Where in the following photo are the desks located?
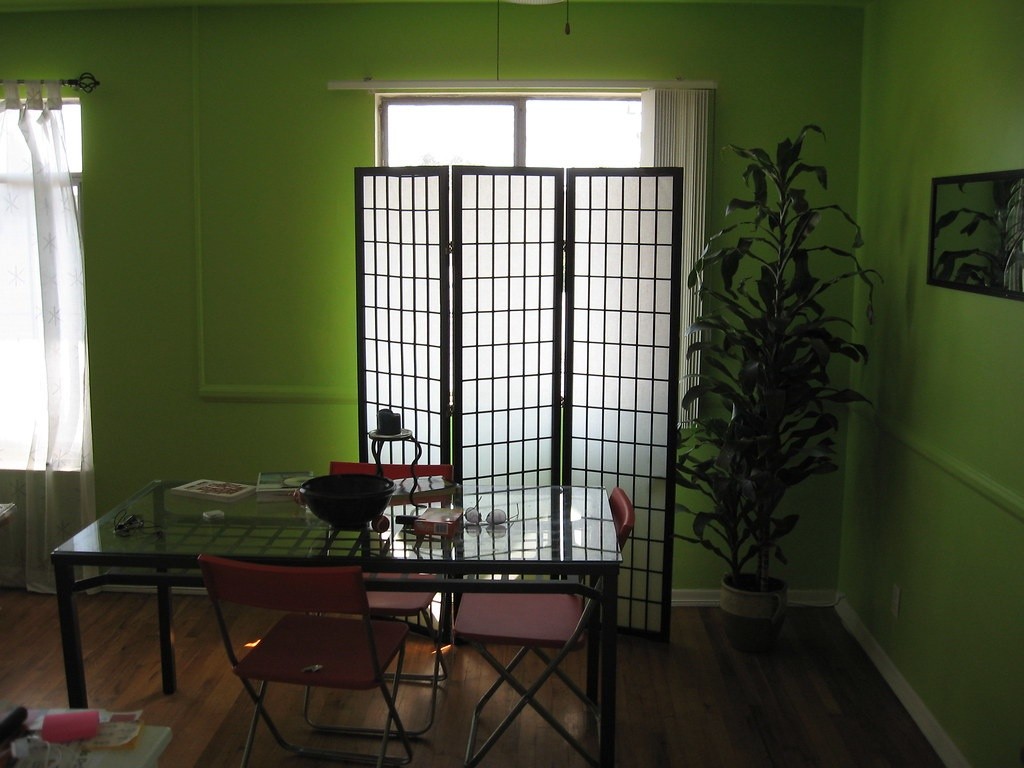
[51,479,623,768]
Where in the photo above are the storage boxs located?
[415,508,463,536]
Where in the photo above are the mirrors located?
[927,168,1024,303]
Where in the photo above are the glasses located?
[463,496,520,525]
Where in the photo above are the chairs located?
[453,488,635,768]
[303,462,455,734]
[199,554,413,768]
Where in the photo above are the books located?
[257,471,314,492]
[170,479,257,503]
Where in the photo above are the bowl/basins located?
[300,472,398,530]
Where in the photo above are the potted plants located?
[669,126,884,655]
